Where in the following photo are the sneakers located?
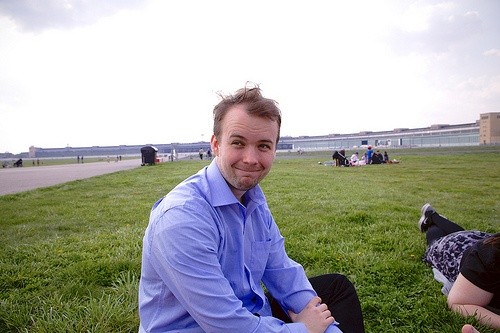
[418,203,442,232]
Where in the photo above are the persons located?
[199,147,204,160]
[138,87,365,333]
[332,145,388,166]
[206,148,212,161]
[418,203,500,333]
